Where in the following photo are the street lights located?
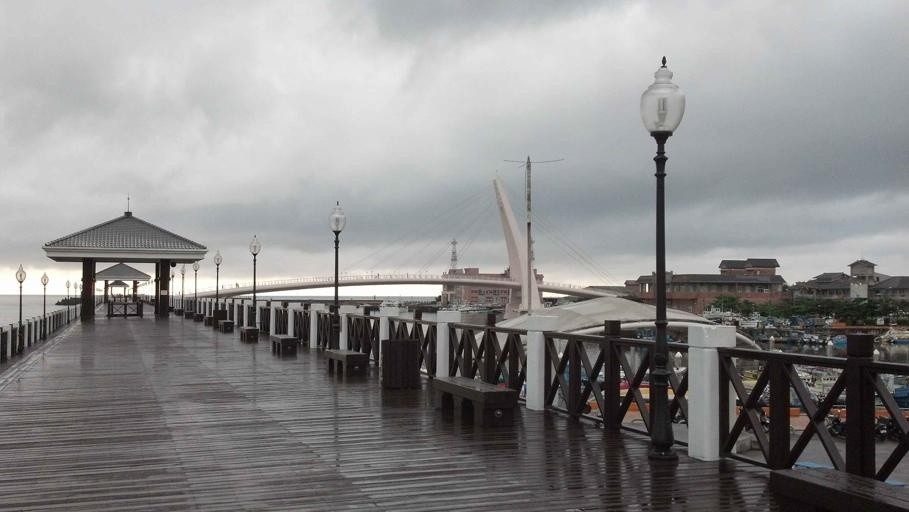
[214,250,222,321]
[330,201,346,350]
[136,279,155,306]
[193,261,200,313]
[66,280,71,324]
[96,288,103,306]
[41,273,48,338]
[638,56,686,450]
[16,264,26,351]
[180,264,187,309]
[249,234,261,327]
[171,270,175,306]
[74,283,82,319]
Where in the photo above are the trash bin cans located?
[382,339,421,388]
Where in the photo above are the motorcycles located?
[875,416,902,442]
[817,395,844,436]
[738,407,771,433]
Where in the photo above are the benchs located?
[431,375,518,428]
[767,467,909,512]
[218,320,234,332]
[168,307,212,325]
[325,348,368,376]
[240,326,259,342]
[271,334,297,355]
